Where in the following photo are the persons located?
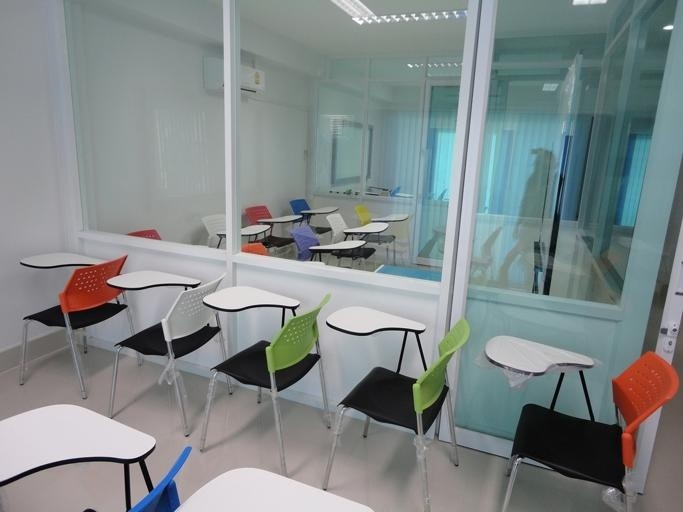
[513,149,556,290]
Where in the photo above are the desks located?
[326,305,427,375]
[202,285,300,329]
[0,403,156,512]
[106,270,201,291]
[485,335,596,422]
[371,213,409,222]
[174,467,375,512]
[20,252,108,269]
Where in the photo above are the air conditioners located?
[202,55,265,95]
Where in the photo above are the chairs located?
[20,254,142,400]
[107,270,232,438]
[127,447,191,512]
[322,306,470,512]
[200,285,331,479]
[126,199,410,271]
[503,336,680,512]
